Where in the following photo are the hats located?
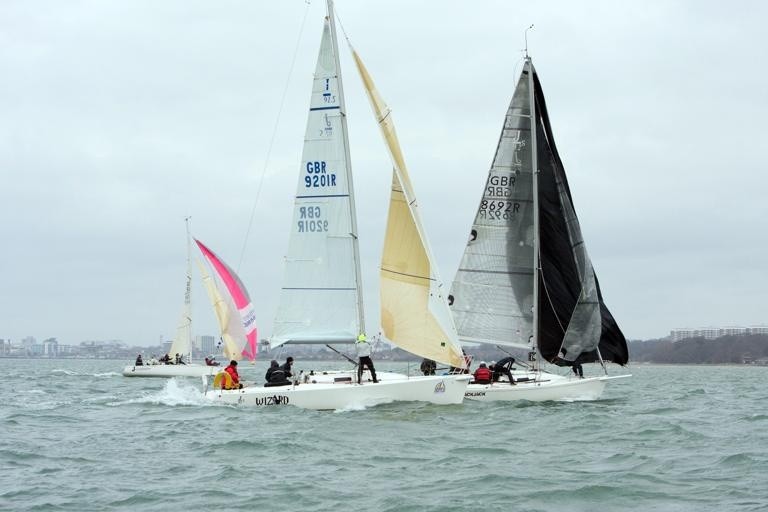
[287,356,294,363]
[357,335,367,341]
[230,360,237,366]
[488,361,497,366]
[480,361,487,367]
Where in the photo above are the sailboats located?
[204,1,475,411]
[463,23,632,404]
[125,216,258,378]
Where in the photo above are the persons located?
[356,332,379,384]
[489,360,499,382]
[420,357,436,376]
[573,363,584,378]
[280,356,294,380]
[222,359,244,390]
[493,356,517,386]
[265,359,287,384]
[134,352,220,366]
[474,361,492,384]
